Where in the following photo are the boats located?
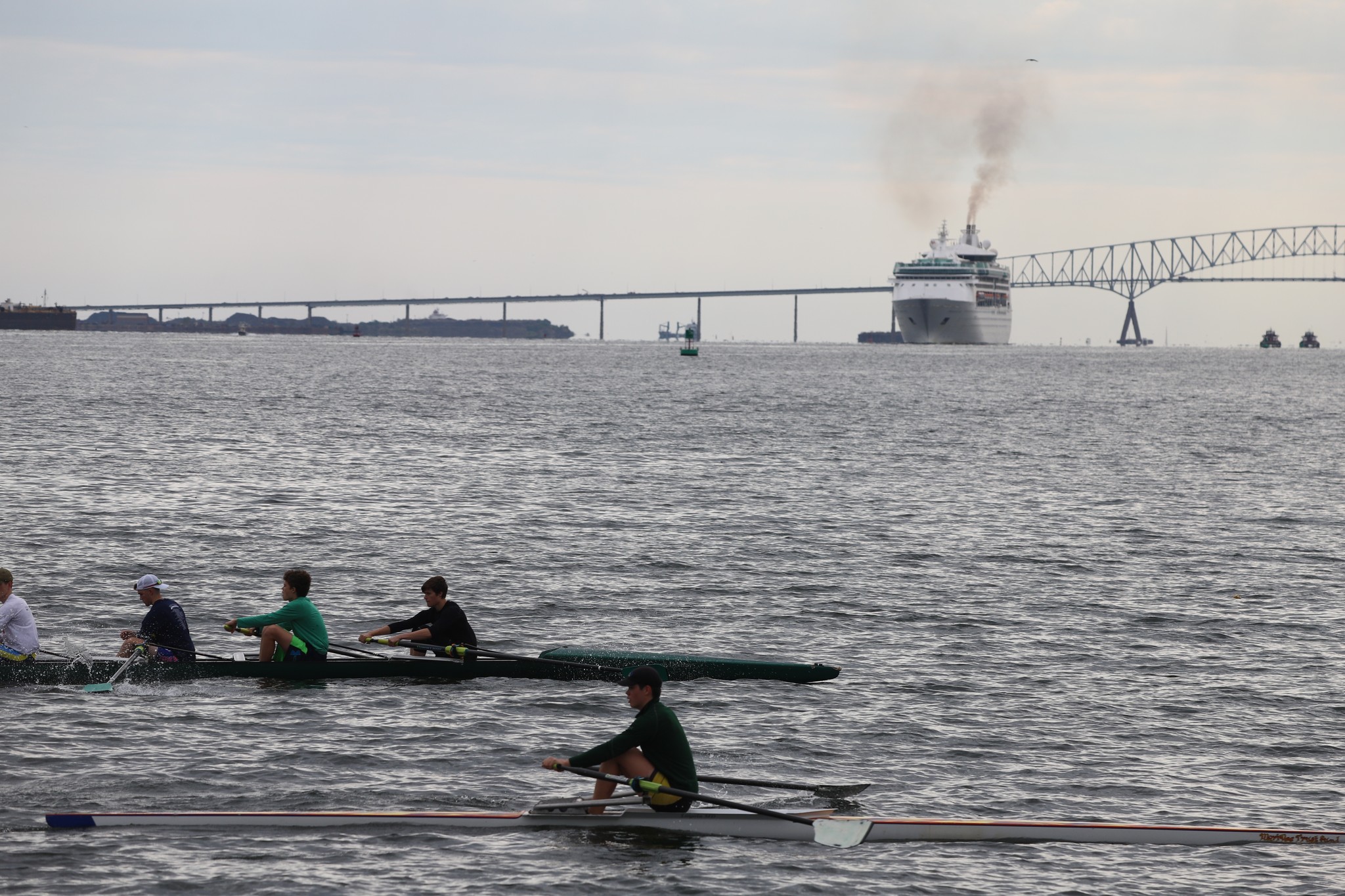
[353,326,360,337]
[1260,330,1281,348]
[1300,331,1320,348]
[957,248,996,261]
[680,329,700,356]
[238,324,247,335]
[0,639,841,683]
[977,292,1008,300]
[45,801,1344,848]
[888,219,1012,344]
[0,298,77,331]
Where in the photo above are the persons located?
[0,567,40,664]
[115,574,196,662]
[542,667,699,815]
[358,576,477,659]
[224,570,329,661]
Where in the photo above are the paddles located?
[223,622,372,658]
[586,763,872,800]
[80,638,150,695]
[365,634,671,684]
[551,757,875,846]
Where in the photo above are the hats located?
[134,574,171,591]
[617,666,664,687]
